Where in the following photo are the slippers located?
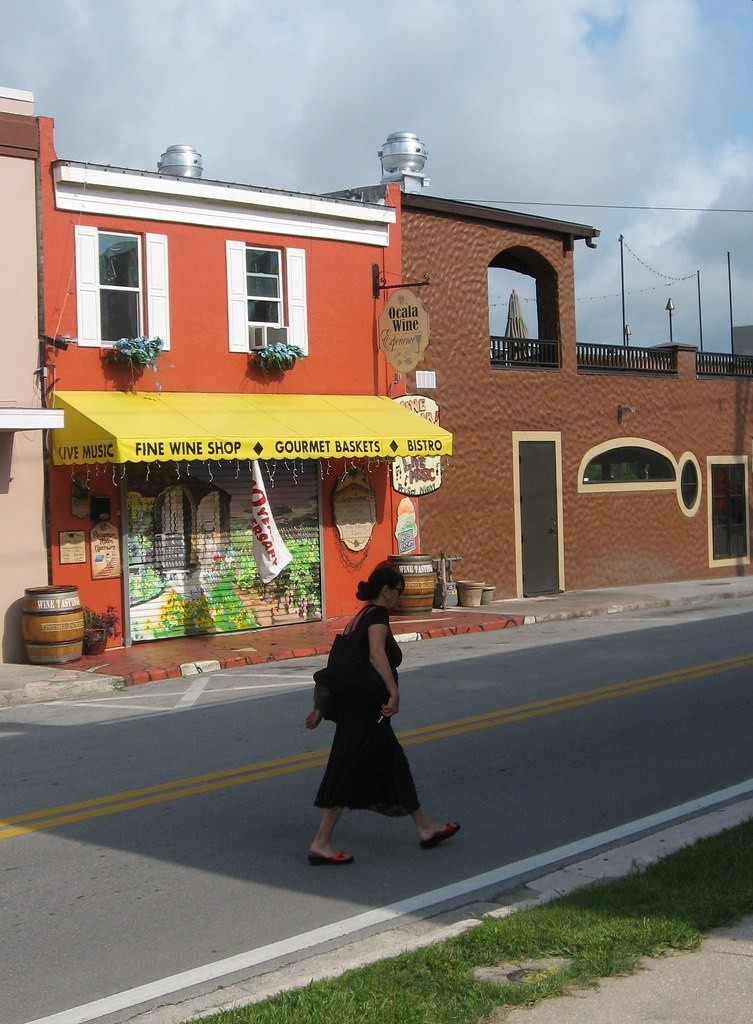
[420,823,460,849]
[308,848,353,866]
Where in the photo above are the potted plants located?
[248,342,304,379]
[82,602,121,655]
[114,335,163,378]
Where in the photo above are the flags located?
[252,460,292,583]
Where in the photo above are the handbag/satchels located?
[313,604,387,721]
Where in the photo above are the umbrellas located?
[503,290,529,338]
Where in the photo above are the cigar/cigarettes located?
[378,716,384,723]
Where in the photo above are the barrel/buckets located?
[388,554,435,616]
[481,585,495,605]
[20,584,84,665]
[456,580,485,607]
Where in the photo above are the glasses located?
[391,587,403,596]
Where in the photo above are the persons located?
[302,562,462,866]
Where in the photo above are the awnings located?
[51,394,452,466]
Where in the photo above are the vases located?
[480,585,495,605]
[455,581,484,608]
